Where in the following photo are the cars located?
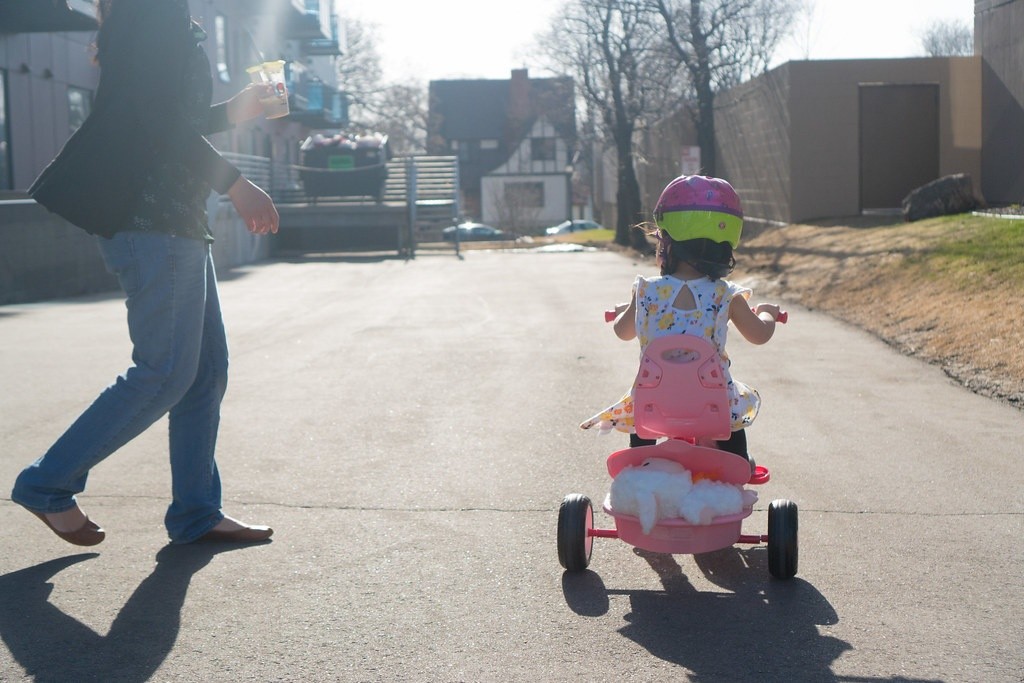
[545,220,602,235]
[444,223,512,242]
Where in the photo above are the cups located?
[247,60,289,118]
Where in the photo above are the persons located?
[10,0,281,548]
[579,175,780,468]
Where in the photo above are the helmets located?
[653,174,743,249]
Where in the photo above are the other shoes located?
[12,495,106,547]
[194,522,274,541]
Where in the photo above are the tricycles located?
[558,302,799,580]
